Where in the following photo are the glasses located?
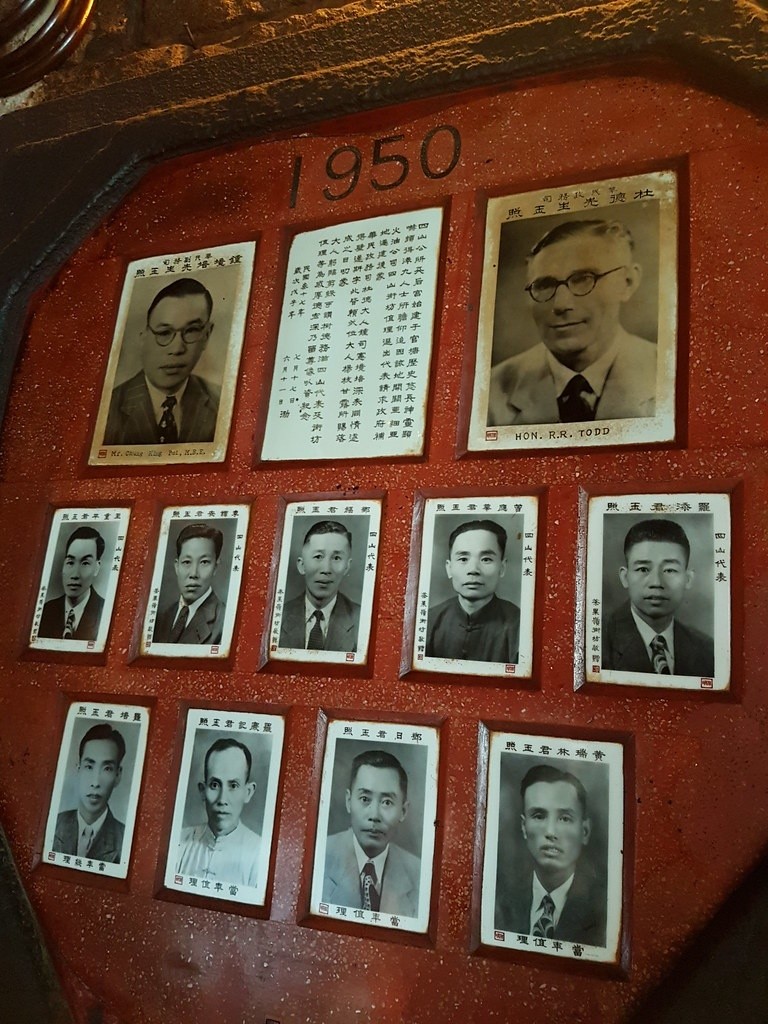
[524,265,624,303]
[147,319,209,346]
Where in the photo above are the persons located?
[153,523,226,645]
[37,526,105,641]
[486,220,656,425]
[322,751,422,918]
[425,520,521,663]
[494,765,607,947]
[602,519,714,679]
[278,521,361,653]
[176,738,261,887]
[102,277,222,445]
[51,722,128,864]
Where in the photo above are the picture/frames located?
[402,486,548,690]
[32,688,156,893]
[296,707,450,948]
[244,194,451,467]
[76,231,258,481]
[569,479,742,703]
[466,718,632,979]
[256,487,387,678]
[10,500,132,666]
[458,153,690,454]
[153,699,294,919]
[125,496,253,669]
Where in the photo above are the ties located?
[307,611,324,649]
[169,606,189,643]
[79,827,92,857]
[158,396,178,444]
[64,609,75,639]
[533,896,554,939]
[362,862,380,912]
[650,636,671,675]
[564,375,593,422]
